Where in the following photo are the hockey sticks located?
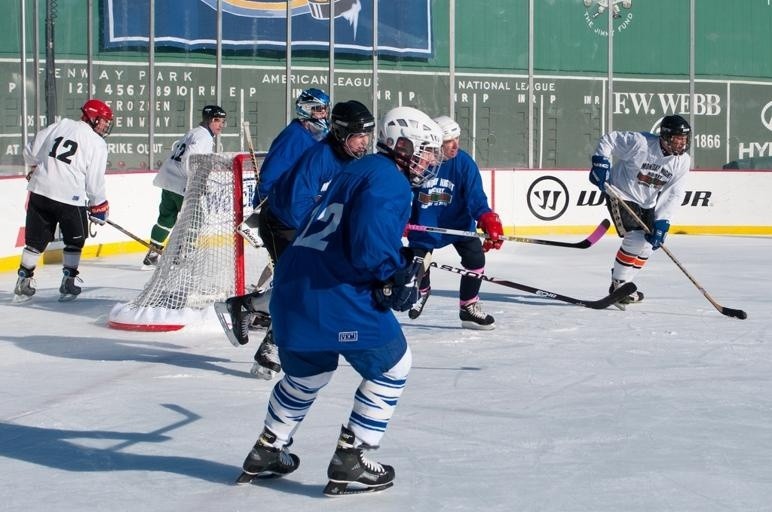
[408,218,611,250]
[602,180,748,320]
[413,256,638,310]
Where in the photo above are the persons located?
[232,106,444,498]
[250,87,333,332]
[13,99,112,300]
[408,113,504,331]
[139,105,226,271]
[214,98,376,383]
[589,114,691,311]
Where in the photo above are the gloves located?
[90,201,110,226]
[644,219,671,251]
[477,212,505,252]
[588,155,611,192]
[377,265,419,313]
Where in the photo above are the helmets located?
[330,100,374,159]
[201,105,226,120]
[81,100,114,139]
[432,114,461,162]
[295,88,330,132]
[660,114,691,156]
[375,105,445,186]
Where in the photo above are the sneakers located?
[608,279,629,305]
[227,292,255,344]
[13,265,36,296]
[143,241,164,265]
[326,423,395,488]
[59,267,82,295]
[242,425,301,477]
[628,291,644,302]
[253,330,282,374]
[458,301,495,326]
[408,287,432,318]
